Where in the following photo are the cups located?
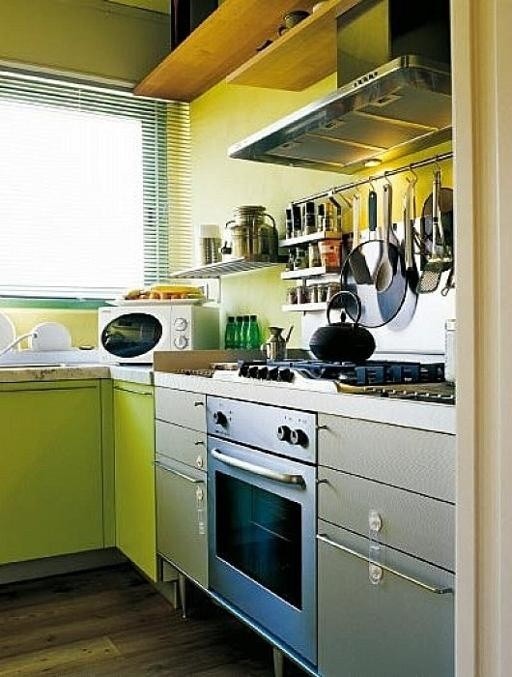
[199,236,221,263]
[442,317,456,384]
[276,10,311,36]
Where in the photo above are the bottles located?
[284,201,340,304]
[223,314,260,349]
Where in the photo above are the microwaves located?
[97,306,220,364]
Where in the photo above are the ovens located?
[206,434,319,675]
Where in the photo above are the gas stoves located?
[205,356,443,463]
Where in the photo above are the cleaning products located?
[223,314,261,352]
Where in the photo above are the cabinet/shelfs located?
[113,379,178,585]
[153,386,208,593]
[317,411,457,677]
[278,230,344,312]
[1,377,105,566]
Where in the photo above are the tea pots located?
[260,324,294,360]
[221,204,280,263]
[309,292,377,360]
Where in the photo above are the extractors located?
[227,0,452,176]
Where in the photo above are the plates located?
[0,312,72,351]
[104,297,214,306]
